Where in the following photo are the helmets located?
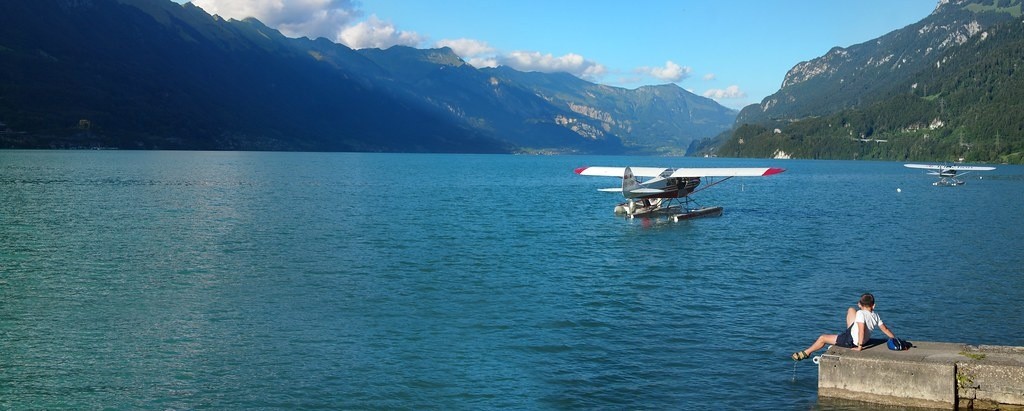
[887,338,904,351]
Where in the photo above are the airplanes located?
[905,164,996,178]
[573,166,788,200]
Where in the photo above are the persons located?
[791,293,895,361]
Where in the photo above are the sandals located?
[792,350,811,361]
